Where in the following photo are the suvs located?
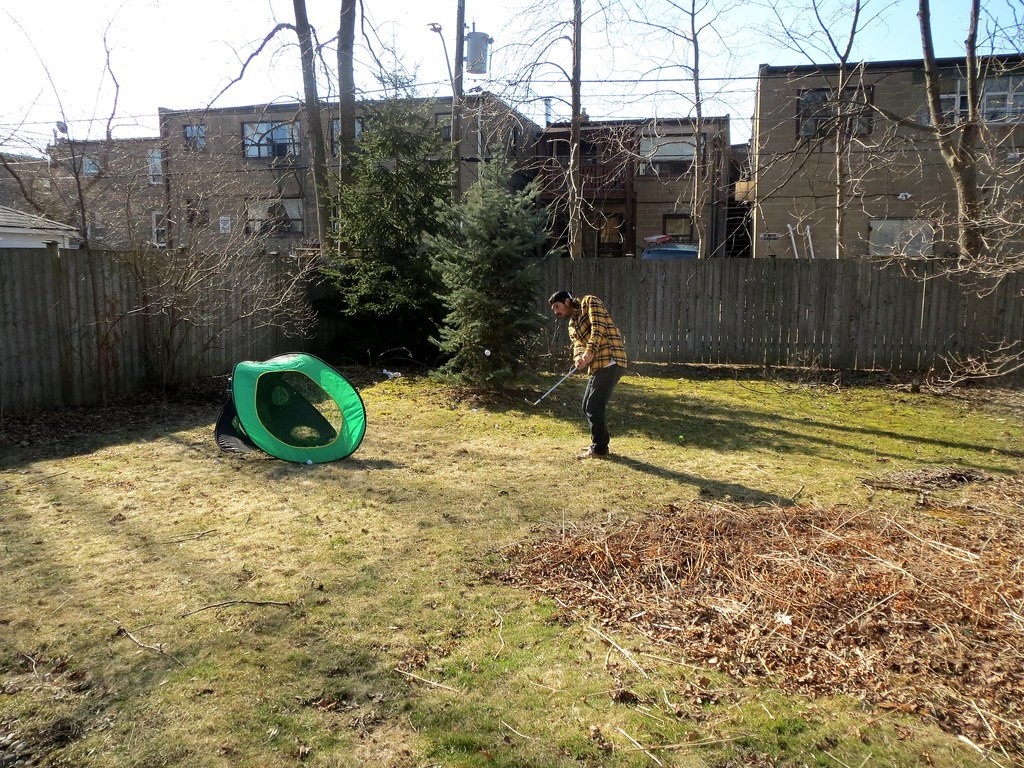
[636,242,699,262]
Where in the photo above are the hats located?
[548,290,573,305]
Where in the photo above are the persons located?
[548,290,628,460]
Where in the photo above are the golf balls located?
[307,460,313,465]
[485,350,490,356]
[679,435,684,439]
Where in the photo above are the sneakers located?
[581,446,590,451]
[577,450,607,460]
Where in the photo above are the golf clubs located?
[523,366,579,406]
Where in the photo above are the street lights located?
[429,25,464,207]
